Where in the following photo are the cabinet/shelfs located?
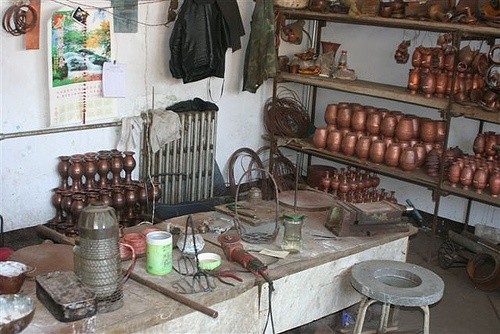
[262,4,500,263]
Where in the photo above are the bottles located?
[48,147,161,238]
[78,201,125,312]
[74,240,81,274]
[278,40,500,205]
[281,213,304,253]
[176,229,205,254]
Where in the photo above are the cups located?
[145,230,173,276]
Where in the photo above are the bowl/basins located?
[0,293,36,334]
[123,232,147,255]
[0,260,28,294]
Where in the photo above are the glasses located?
[167,0,179,22]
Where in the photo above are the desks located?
[7,197,418,334]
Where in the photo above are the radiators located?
[139,109,219,206]
